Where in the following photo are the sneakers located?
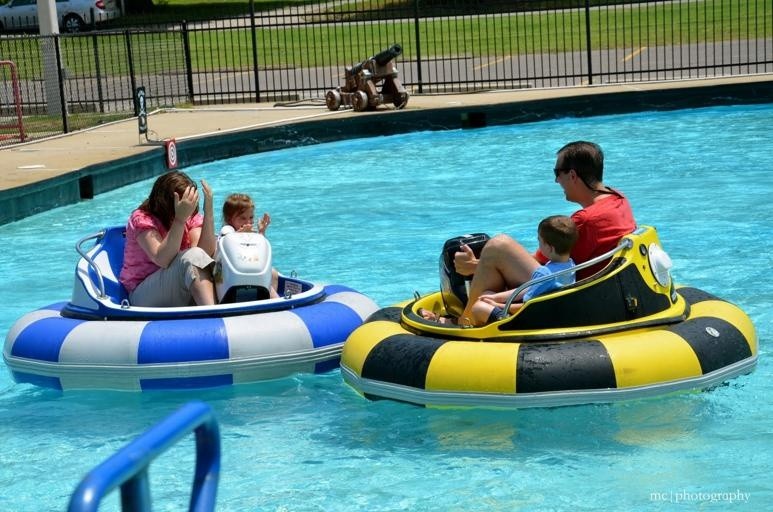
[418,308,451,325]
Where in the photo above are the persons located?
[117,168,220,307]
[466,213,578,328]
[213,192,281,300]
[415,140,637,330]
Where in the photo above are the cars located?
[0,1,121,35]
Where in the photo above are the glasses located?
[554,169,570,177]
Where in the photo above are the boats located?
[1,224,381,393]
[333,225,760,417]
